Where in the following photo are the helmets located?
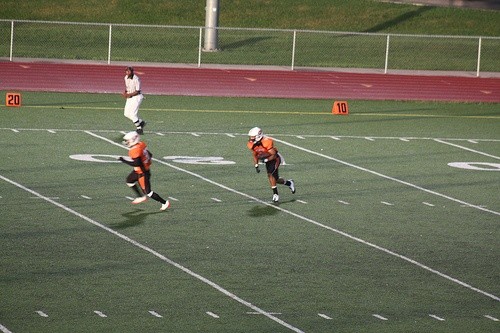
[121,131,140,148]
[248,126,264,144]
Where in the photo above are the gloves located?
[255,163,260,173]
[260,158,268,163]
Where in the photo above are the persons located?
[122,62,147,135]
[117,131,171,211]
[246,127,296,205]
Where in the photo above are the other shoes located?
[135,119,144,135]
[160,200,171,211]
[273,194,279,201]
[131,194,148,205]
[288,179,295,193]
